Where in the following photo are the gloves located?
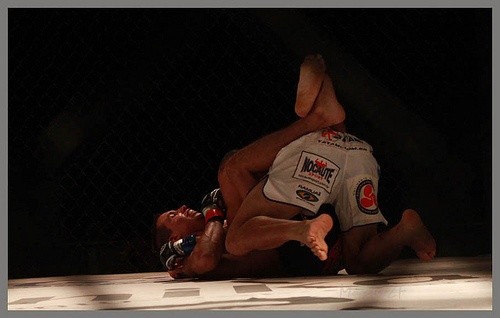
[200,189,227,223]
[160,235,196,270]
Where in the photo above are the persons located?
[149,55,346,277]
[216,128,437,275]
